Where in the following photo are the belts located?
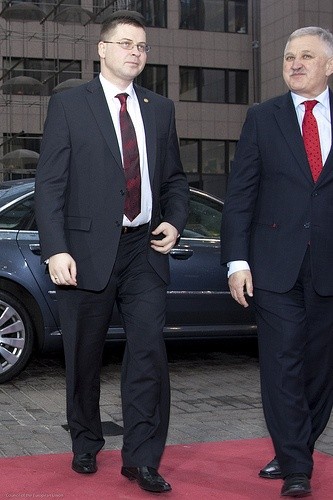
[121,223,149,235]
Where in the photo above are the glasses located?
[100,40,151,53]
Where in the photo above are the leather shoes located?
[72,453,97,474]
[260,457,284,479]
[121,464,171,492]
[281,474,311,494]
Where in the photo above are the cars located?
[0,177,260,385]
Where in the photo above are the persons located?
[220,26,333,498]
[34,10,191,492]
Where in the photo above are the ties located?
[302,100,323,184]
[114,93,141,222]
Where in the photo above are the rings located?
[55,277,58,280]
